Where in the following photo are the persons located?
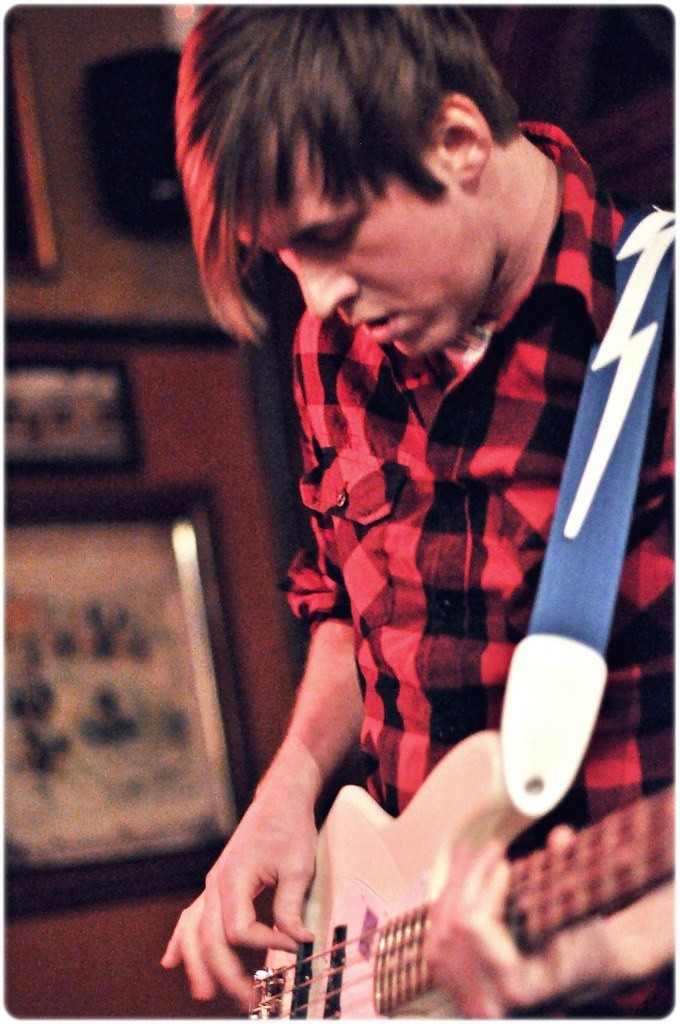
[161,4,675,1020]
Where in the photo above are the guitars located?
[249,726,677,1021]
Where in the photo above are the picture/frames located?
[4,345,145,476]
[5,476,261,919]
